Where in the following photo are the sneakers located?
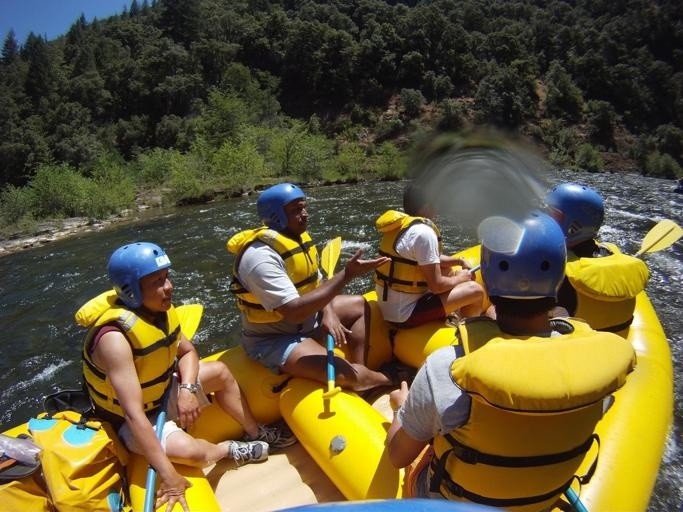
[384,365,416,387]
[225,440,269,465]
[243,424,299,447]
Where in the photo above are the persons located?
[543,183,649,338]
[374,188,483,328]
[75,242,297,512]
[227,183,393,392]
[385,209,635,512]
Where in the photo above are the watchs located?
[180,383,201,393]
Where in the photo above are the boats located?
[0,219,683,512]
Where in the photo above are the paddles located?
[634,219,683,257]
[321,238,340,400]
[140,302,203,511]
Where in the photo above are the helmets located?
[480,210,567,302]
[256,184,307,230]
[544,183,604,242]
[107,241,173,312]
